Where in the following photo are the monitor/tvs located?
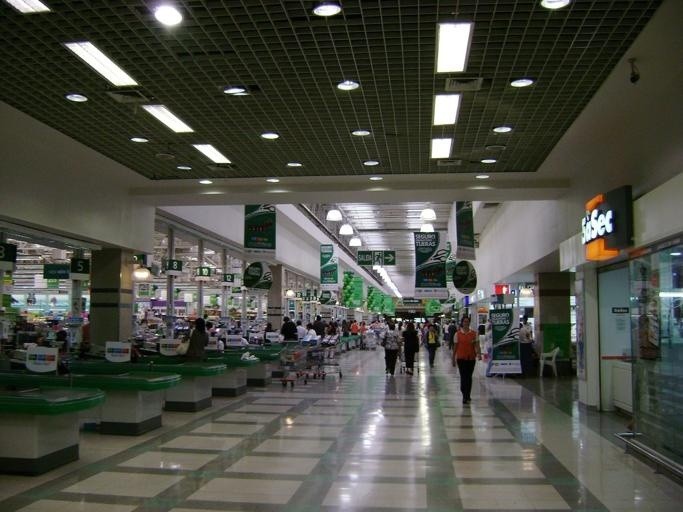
[174,328,190,342]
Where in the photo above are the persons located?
[205,321,219,337]
[451,316,480,404]
[262,313,458,376]
[522,313,535,344]
[519,322,529,348]
[229,329,250,345]
[184,317,209,358]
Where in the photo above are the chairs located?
[539,346,559,378]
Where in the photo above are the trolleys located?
[400,346,419,374]
[362,331,377,351]
[280,335,341,387]
